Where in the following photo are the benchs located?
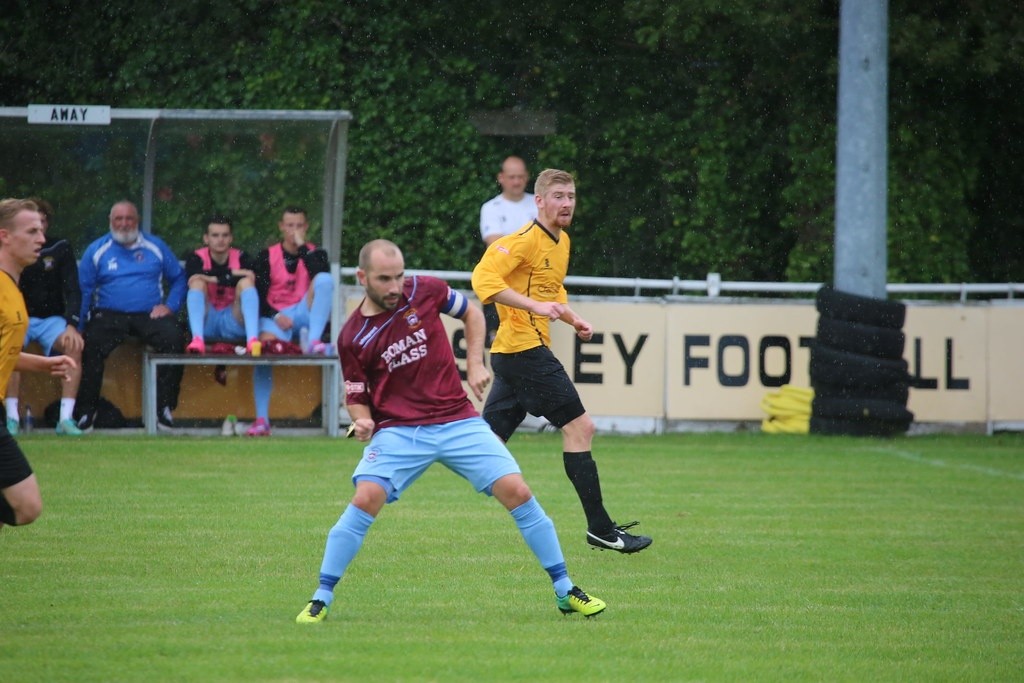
[139,347,346,439]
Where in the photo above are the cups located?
[252,341,261,356]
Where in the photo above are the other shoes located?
[539,423,561,433]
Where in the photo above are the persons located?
[77,199,187,435]
[246,206,334,438]
[480,154,558,433]
[471,168,653,554]
[4,199,84,435]
[185,215,262,356]
[0,199,77,528]
[295,239,607,623]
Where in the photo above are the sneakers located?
[586,521,653,554]
[249,418,271,435]
[555,586,606,618]
[306,340,326,356]
[77,406,98,433]
[188,337,205,354]
[245,338,261,354]
[158,408,184,434]
[6,417,18,435]
[296,600,328,624]
[56,419,82,435]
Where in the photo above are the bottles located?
[24,406,33,436]
